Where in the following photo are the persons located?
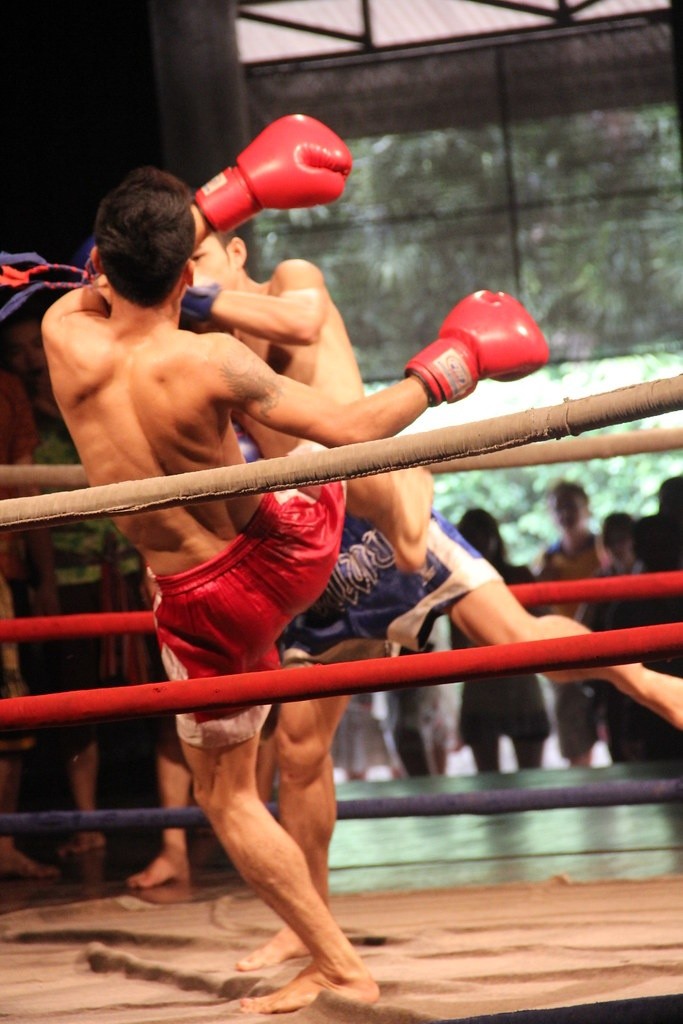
[41,112,551,1015]
[0,226,683,968]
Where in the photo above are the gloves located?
[197,113,353,234]
[405,290,550,409]
